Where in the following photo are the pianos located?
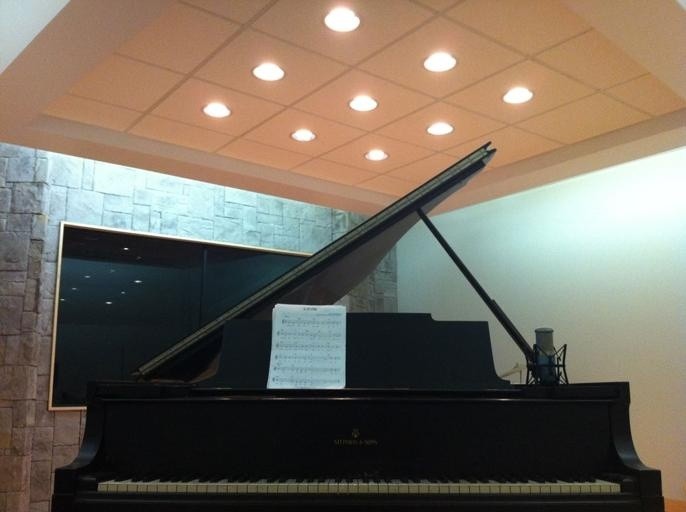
[50,142,666,509]
